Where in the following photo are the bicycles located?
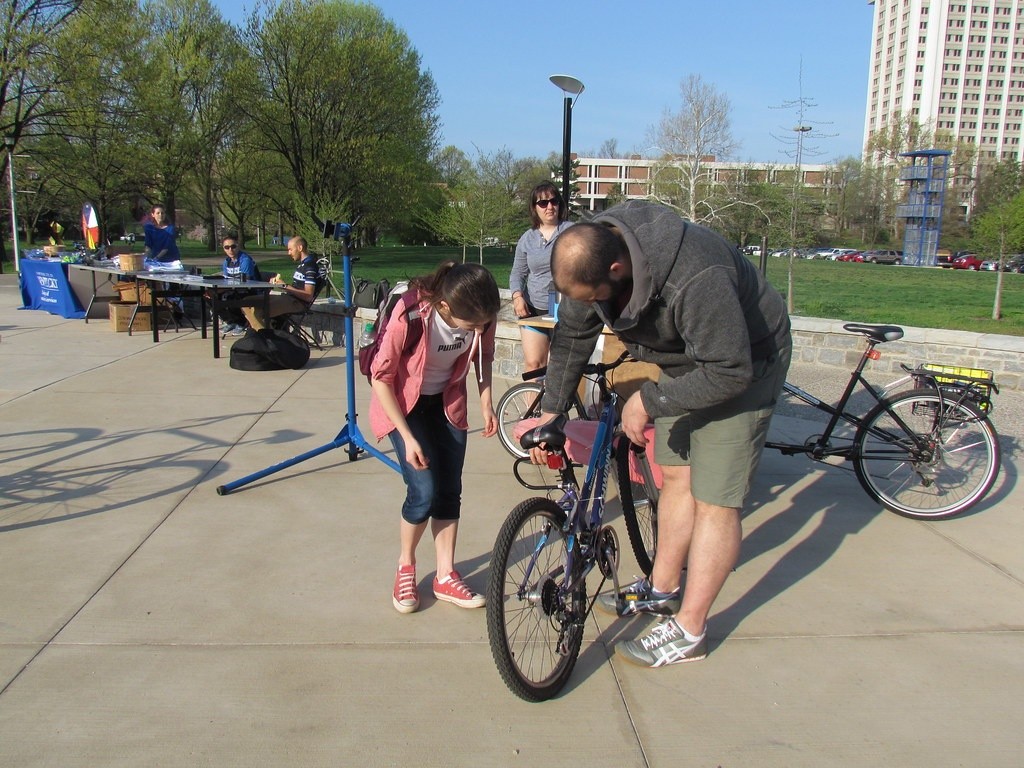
[486,350,660,703]
[763,322,1001,521]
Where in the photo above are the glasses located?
[535,197,560,209]
[225,245,236,249]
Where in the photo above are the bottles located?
[358,323,379,350]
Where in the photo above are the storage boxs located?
[116,279,152,304]
[108,300,153,331]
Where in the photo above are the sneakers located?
[615,616,708,669]
[435,571,485,608]
[599,575,681,617]
[232,321,248,336]
[220,322,237,334]
[393,558,420,612]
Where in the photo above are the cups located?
[240,273,245,282]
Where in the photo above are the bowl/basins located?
[57,252,80,263]
[43,245,67,257]
[22,249,52,262]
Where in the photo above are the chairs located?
[150,264,197,334]
[270,259,330,351]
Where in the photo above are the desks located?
[71,258,189,339]
[138,272,287,360]
[16,258,122,321]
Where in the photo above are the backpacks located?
[359,281,422,386]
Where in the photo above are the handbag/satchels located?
[354,279,390,309]
[229,328,310,372]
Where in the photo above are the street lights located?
[549,74,585,221]
[4,130,20,272]
[793,126,812,237]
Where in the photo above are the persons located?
[143,204,182,315]
[243,237,321,342]
[528,198,792,668]
[210,236,261,334]
[369,263,498,614]
[508,180,581,441]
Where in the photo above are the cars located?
[743,246,828,260]
[855,250,875,263]
[980,255,1014,271]
[863,250,903,265]
[484,236,499,247]
[817,248,856,260]
[272,230,290,245]
[952,254,984,271]
[837,250,863,262]
[825,250,846,261]
[1006,254,1024,274]
[120,233,135,241]
[937,251,973,268]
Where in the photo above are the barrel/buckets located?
[119,253,144,271]
